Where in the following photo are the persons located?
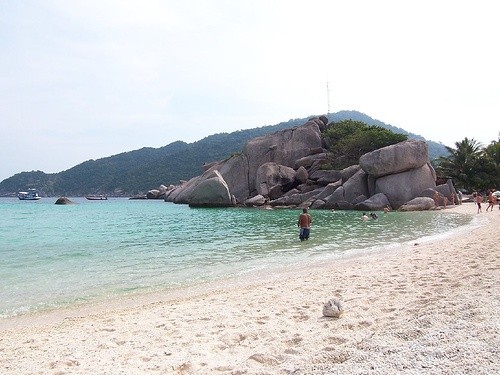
[450,191,454,205]
[442,195,448,207]
[386,204,392,210]
[361,212,368,221]
[370,213,378,220]
[383,207,390,213]
[475,194,483,214]
[458,191,462,205]
[331,208,335,213]
[298,209,311,241]
[472,190,477,205]
[486,192,495,211]
[432,190,439,207]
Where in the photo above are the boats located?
[86,197,108,200]
[16,188,42,200]
